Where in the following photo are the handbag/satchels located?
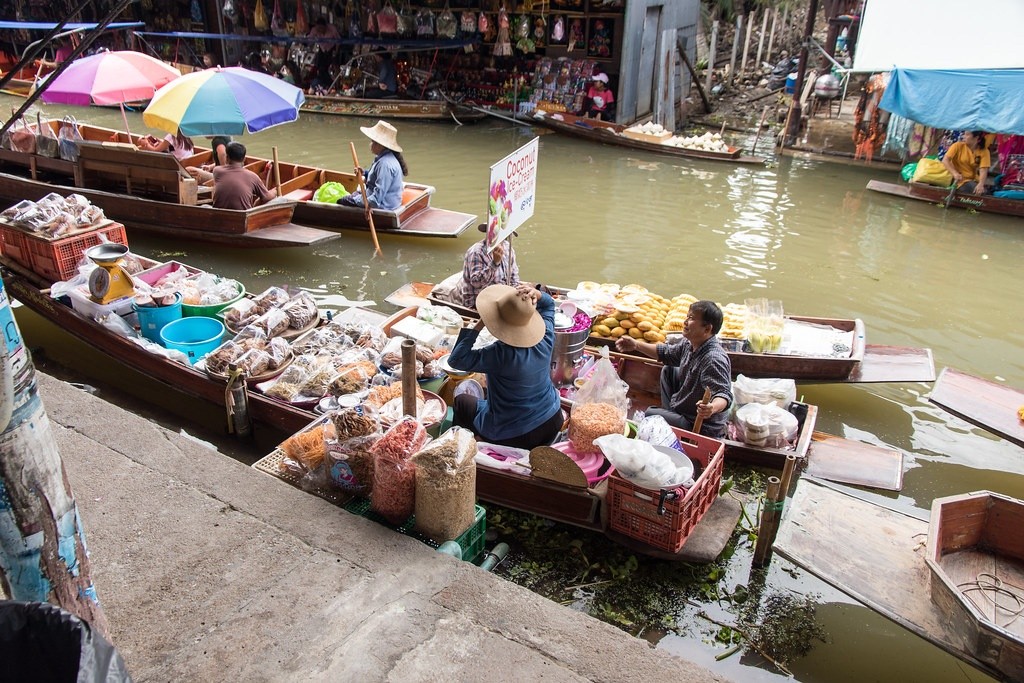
[253,0,311,36]
[346,0,496,39]
[2,106,84,162]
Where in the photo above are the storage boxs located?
[606,425,725,555]
[0,222,208,320]
[623,128,672,142]
[388,315,444,349]
[249,449,487,567]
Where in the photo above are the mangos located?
[589,293,671,341]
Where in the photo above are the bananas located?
[748,331,782,353]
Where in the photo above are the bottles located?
[448,68,537,115]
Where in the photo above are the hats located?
[478,222,519,239]
[371,46,391,59]
[360,120,403,152]
[591,73,609,83]
[475,284,547,348]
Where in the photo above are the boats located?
[0,145,343,247]
[522,108,770,165]
[4,116,484,238]
[771,473,1024,683]
[0,48,498,118]
[385,271,935,384]
[865,177,1024,217]
[929,367,1024,449]
[0,199,908,564]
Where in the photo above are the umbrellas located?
[37,48,181,144]
[142,65,306,159]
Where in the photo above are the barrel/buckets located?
[785,75,796,94]
[374,352,446,393]
[832,66,848,97]
[549,441,615,490]
[616,445,694,536]
[130,292,183,348]
[159,316,226,366]
[550,301,592,388]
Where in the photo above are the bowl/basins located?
[319,393,361,413]
[382,388,447,439]
[182,278,245,321]
[558,301,578,317]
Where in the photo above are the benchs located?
[179,150,313,207]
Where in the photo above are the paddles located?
[349,141,380,248]
[688,386,711,446]
[271,145,282,198]
[438,87,532,126]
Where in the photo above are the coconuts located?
[630,120,729,152]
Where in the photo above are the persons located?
[307,16,341,95]
[942,131,991,196]
[586,73,614,121]
[336,120,408,211]
[447,284,564,451]
[35,34,77,69]
[355,45,398,99]
[213,143,277,210]
[615,301,733,443]
[194,53,218,72]
[184,135,232,187]
[461,221,519,310]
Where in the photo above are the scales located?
[437,353,483,408]
[85,242,136,305]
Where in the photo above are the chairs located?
[810,71,849,120]
